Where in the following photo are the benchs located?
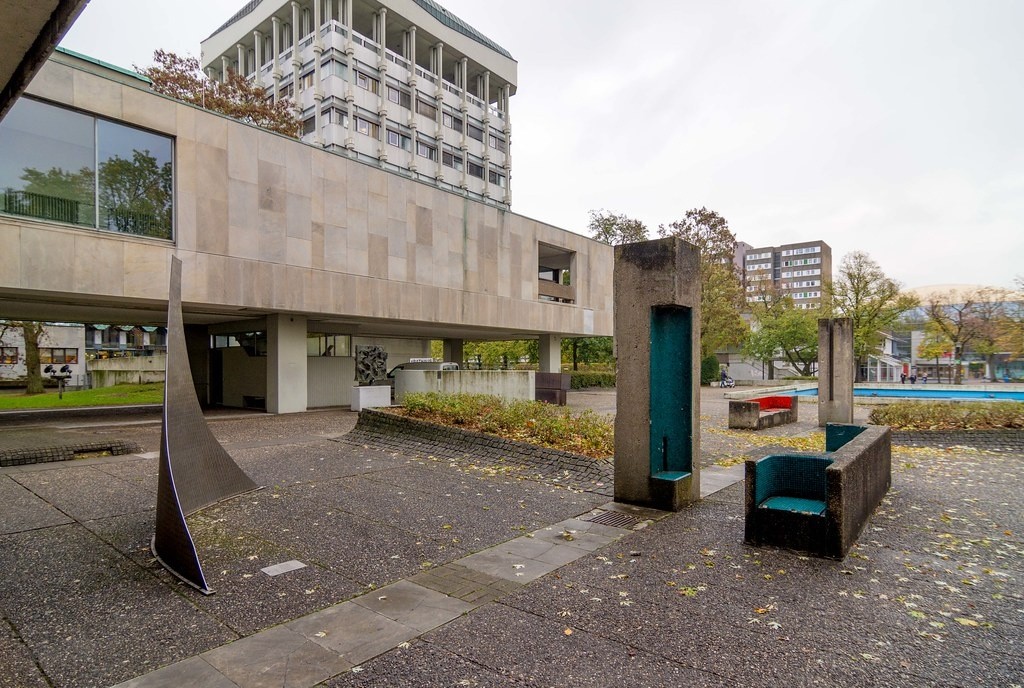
[744,422,891,561]
[729,395,798,431]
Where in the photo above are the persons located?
[910,374,917,384]
[922,373,927,384]
[720,370,728,388]
[901,371,906,384]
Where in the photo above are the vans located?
[386,361,459,378]
